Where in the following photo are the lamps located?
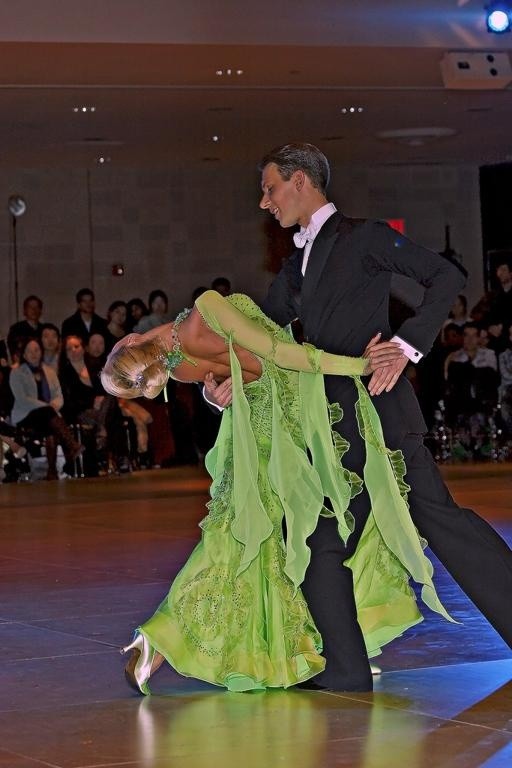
[483,0,512,34]
[8,195,27,324]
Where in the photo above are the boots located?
[48,414,86,461]
[38,444,59,482]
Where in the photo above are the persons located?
[98,286,467,695]
[1,279,230,481]
[406,269,510,463]
[198,140,511,693]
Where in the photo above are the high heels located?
[117,626,157,699]
